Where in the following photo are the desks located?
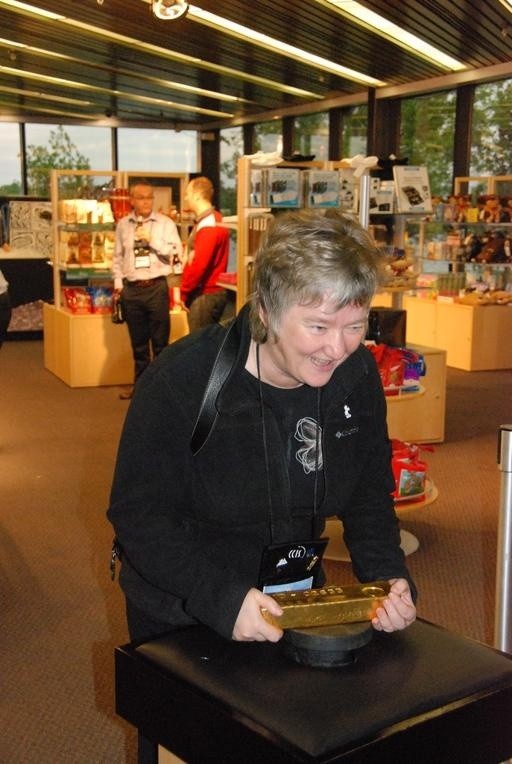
[116,616,512,764]
[320,475,439,562]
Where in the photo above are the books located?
[246,162,434,218]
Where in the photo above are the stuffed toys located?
[379,194,512,308]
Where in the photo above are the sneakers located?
[120,391,132,400]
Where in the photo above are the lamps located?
[151,0,189,24]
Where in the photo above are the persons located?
[105,200,419,643]
[0,202,14,352]
[110,180,183,400]
[178,177,230,331]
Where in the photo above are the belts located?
[135,280,155,288]
